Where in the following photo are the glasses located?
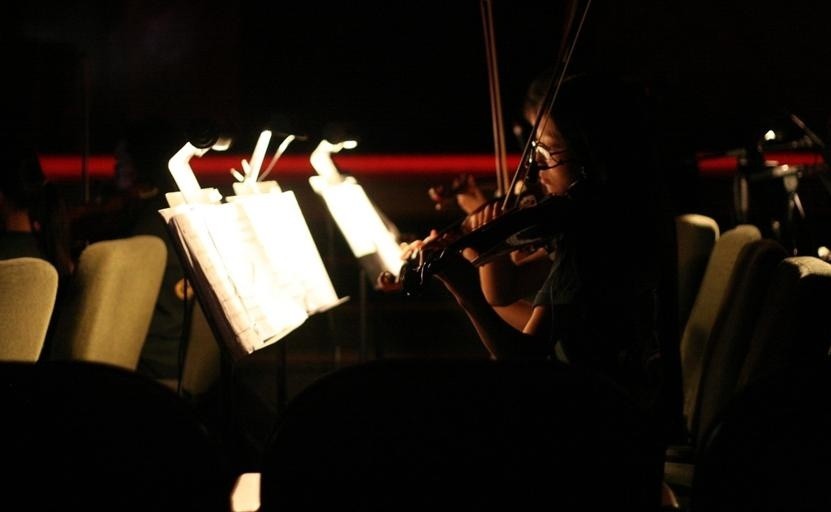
[526,141,569,166]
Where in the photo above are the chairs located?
[42,232,170,376]
[673,213,831,511]
[175,297,225,398]
[1,256,60,364]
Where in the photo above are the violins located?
[377,178,584,298]
